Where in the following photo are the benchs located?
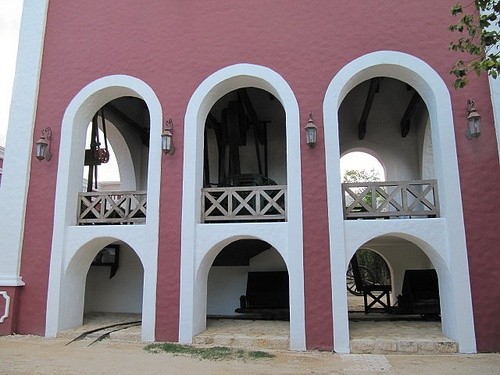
[399,269,440,315]
[236,271,289,314]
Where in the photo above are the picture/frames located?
[91,244,119,266]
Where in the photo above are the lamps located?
[161,119,175,155]
[305,112,317,149]
[465,98,481,140]
[36,127,52,161]
